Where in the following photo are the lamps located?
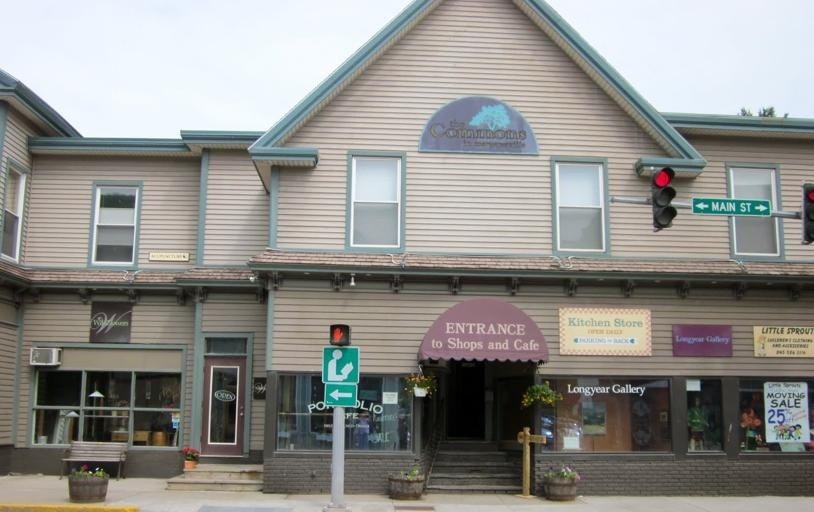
[87,390,104,398]
[64,410,79,418]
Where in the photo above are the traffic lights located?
[329,324,350,346]
[802,183,814,242]
[651,167,677,229]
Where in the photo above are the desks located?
[108,430,152,448]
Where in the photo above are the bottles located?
[740,442,745,452]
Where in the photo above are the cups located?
[289,443,293,449]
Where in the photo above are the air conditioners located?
[29,346,61,366]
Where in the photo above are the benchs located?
[56,439,129,480]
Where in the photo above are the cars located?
[538,413,585,450]
[540,386,653,450]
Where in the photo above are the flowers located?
[520,381,564,406]
[400,375,440,398]
[71,463,108,478]
[182,446,201,461]
[544,464,582,481]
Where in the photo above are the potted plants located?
[385,463,425,499]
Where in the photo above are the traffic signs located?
[692,198,772,218]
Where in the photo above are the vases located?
[183,458,197,470]
[412,387,427,398]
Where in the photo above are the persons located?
[687,396,711,451]
